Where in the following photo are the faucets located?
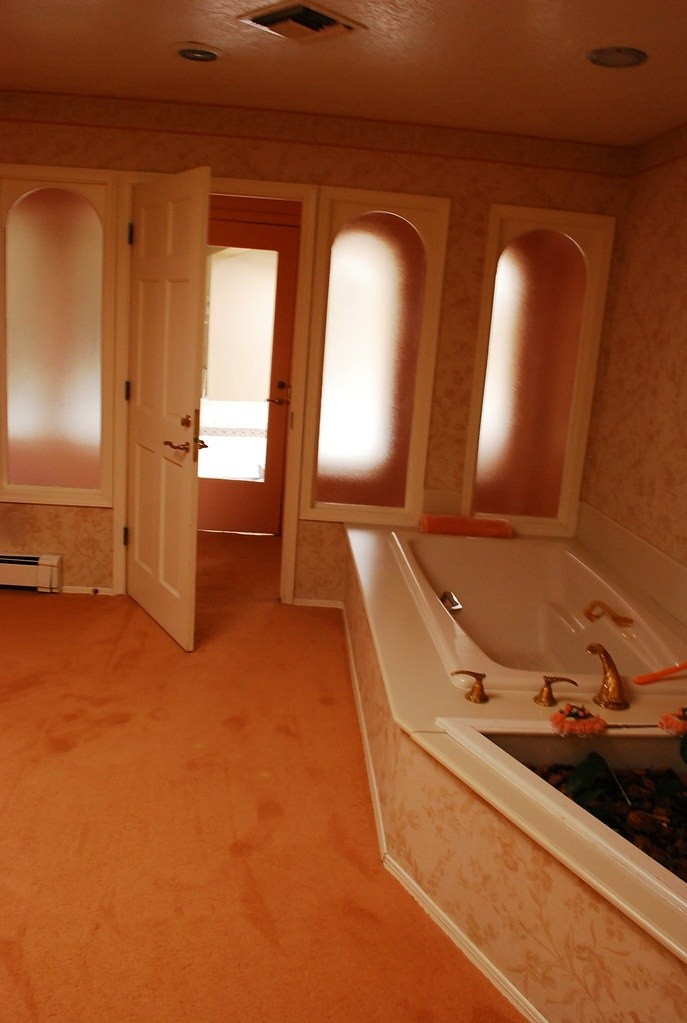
[586,642,630,711]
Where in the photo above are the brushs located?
[634,662,687,685]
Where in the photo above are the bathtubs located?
[340,524,687,733]
